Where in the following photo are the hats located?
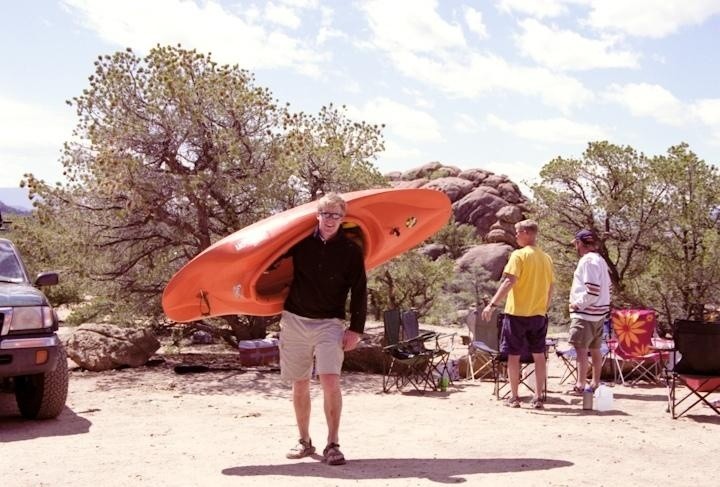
[570,230,594,245]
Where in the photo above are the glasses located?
[514,231,524,234]
[319,210,342,220]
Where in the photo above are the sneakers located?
[504,396,520,407]
[564,386,584,395]
[586,385,599,396]
[530,398,544,409]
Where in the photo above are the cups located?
[439,376,448,391]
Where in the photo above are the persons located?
[480,218,557,409]
[264,192,368,465]
[568,229,612,393]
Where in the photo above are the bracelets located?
[573,303,578,310]
[486,300,498,312]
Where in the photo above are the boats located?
[163,188,452,323]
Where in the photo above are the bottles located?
[582,386,592,409]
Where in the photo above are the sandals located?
[287,438,314,458]
[324,443,346,465]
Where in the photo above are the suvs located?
[0,214,69,419]
